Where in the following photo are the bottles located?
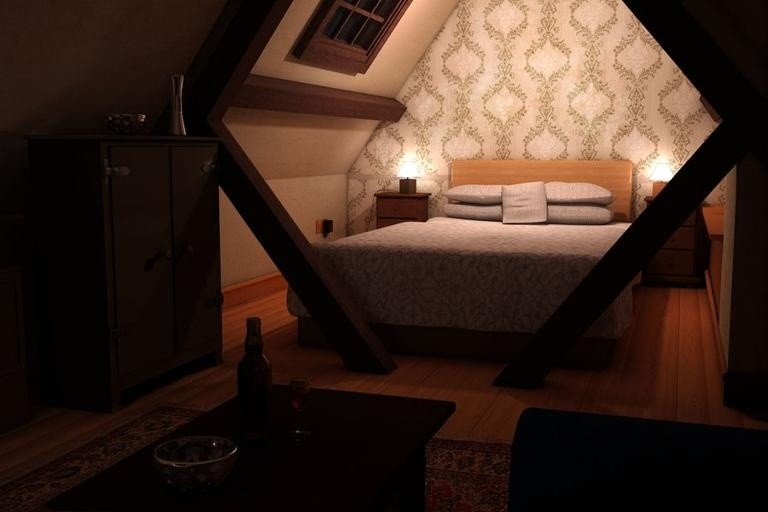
[285,376,309,417]
[168,73,188,138]
[236,316,273,442]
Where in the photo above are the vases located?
[170,73,186,136]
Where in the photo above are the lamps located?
[648,164,674,196]
[396,157,425,193]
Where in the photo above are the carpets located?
[0,406,512,512]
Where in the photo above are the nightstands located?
[374,193,432,229]
[640,196,705,288]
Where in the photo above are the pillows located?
[444,181,614,224]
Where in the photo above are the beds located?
[286,160,633,371]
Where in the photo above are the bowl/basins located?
[103,111,153,135]
[154,436,238,484]
